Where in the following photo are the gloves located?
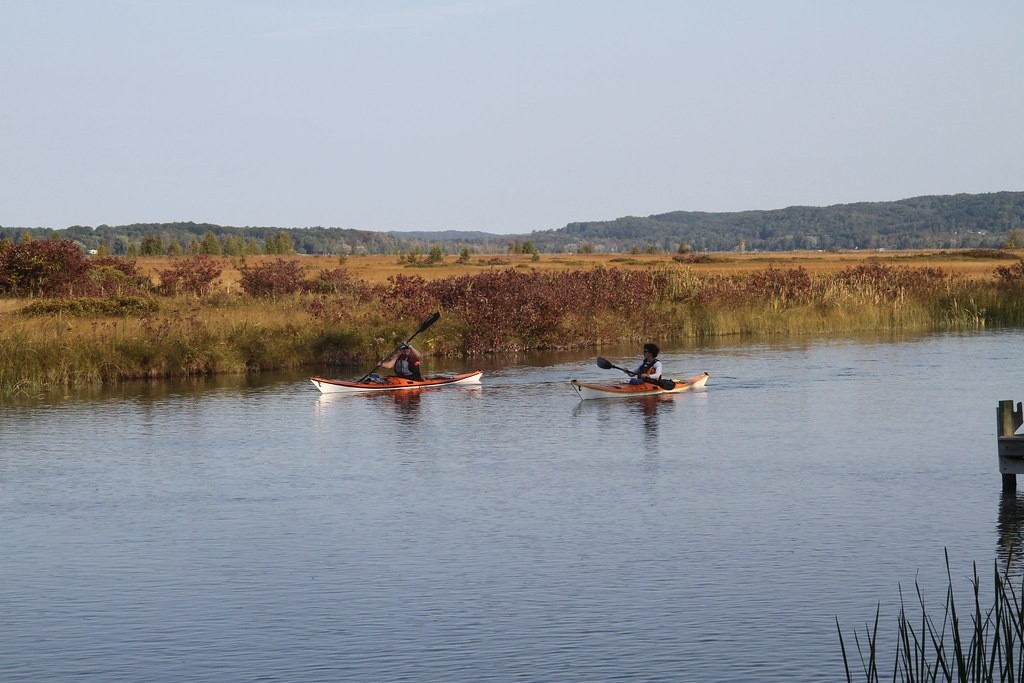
[375,355,383,367]
[398,341,412,349]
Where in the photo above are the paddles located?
[596,355,676,391]
[353,312,441,384]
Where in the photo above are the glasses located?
[644,350,648,353]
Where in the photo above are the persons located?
[377,340,422,380]
[623,344,662,385]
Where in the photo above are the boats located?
[309,369,484,394]
[571,372,709,401]
